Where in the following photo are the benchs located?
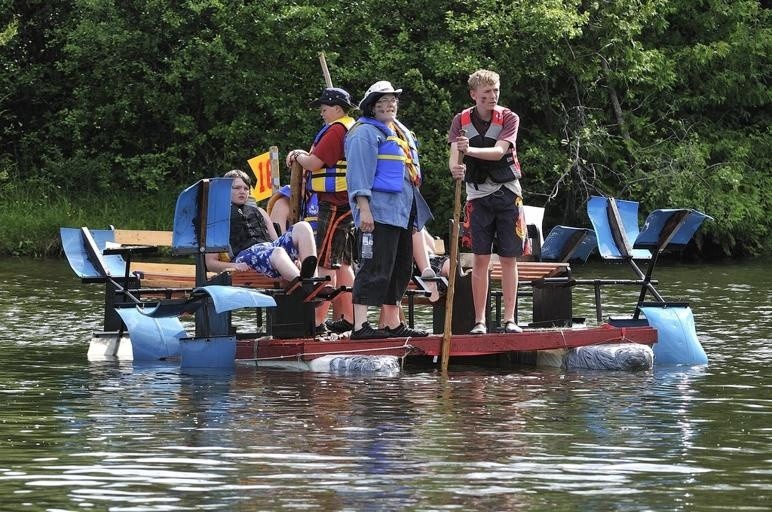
[210,262,570,334]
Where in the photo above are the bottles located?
[361,232,374,259]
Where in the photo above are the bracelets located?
[294,152,301,162]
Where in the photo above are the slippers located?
[420,267,440,302]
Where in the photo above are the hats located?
[308,87,360,111]
[358,80,403,111]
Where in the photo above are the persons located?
[204,169,336,297]
[268,162,322,239]
[411,226,450,302]
[286,88,359,333]
[342,80,429,339]
[446,69,532,334]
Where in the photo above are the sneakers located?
[314,314,353,342]
[350,322,390,340]
[505,321,523,334]
[300,255,317,294]
[469,322,487,334]
[384,320,429,337]
[313,282,337,295]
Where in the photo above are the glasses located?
[375,98,400,105]
